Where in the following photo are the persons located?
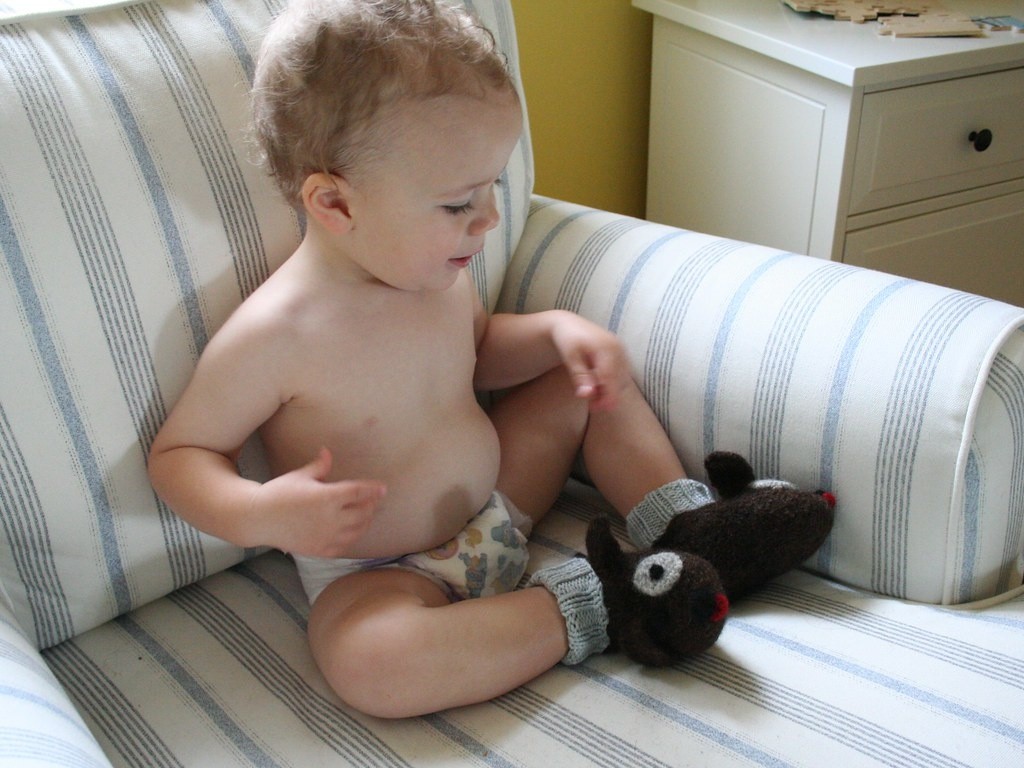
[147,0,834,719]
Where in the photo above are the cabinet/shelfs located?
[631,0,1024,308]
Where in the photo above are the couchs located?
[0,0,1024,768]
